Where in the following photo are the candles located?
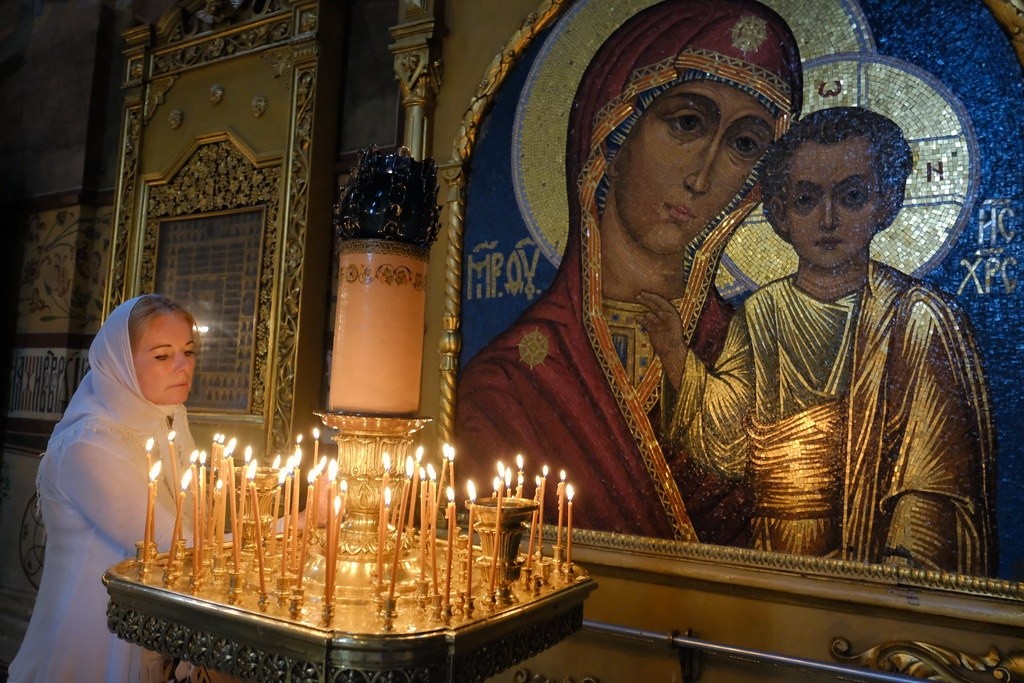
[142,426,574,605]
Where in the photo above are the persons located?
[6,294,220,683]
